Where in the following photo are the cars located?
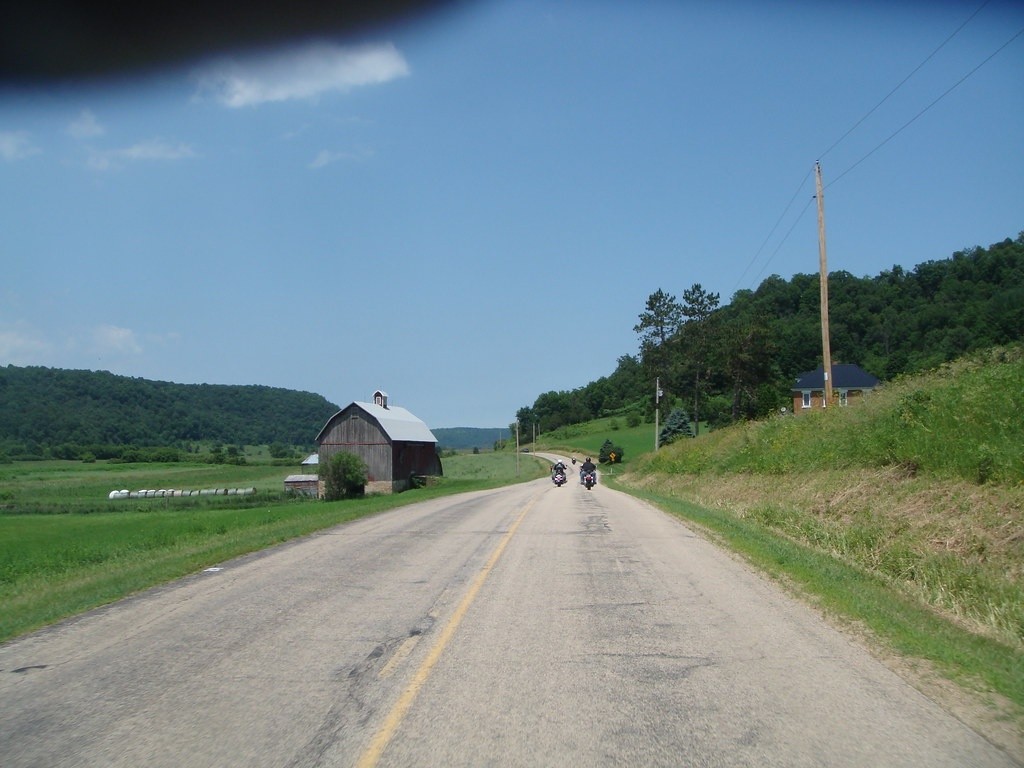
[522,448,529,453]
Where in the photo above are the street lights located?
[655,387,663,453]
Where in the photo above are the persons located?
[552,460,567,484]
[571,456,576,465]
[580,457,597,485]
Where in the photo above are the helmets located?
[586,457,591,461]
[558,460,562,462]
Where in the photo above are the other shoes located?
[581,481,584,484]
[594,482,596,484]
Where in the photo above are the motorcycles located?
[550,464,566,486]
[580,465,597,490]
[573,460,576,465]
